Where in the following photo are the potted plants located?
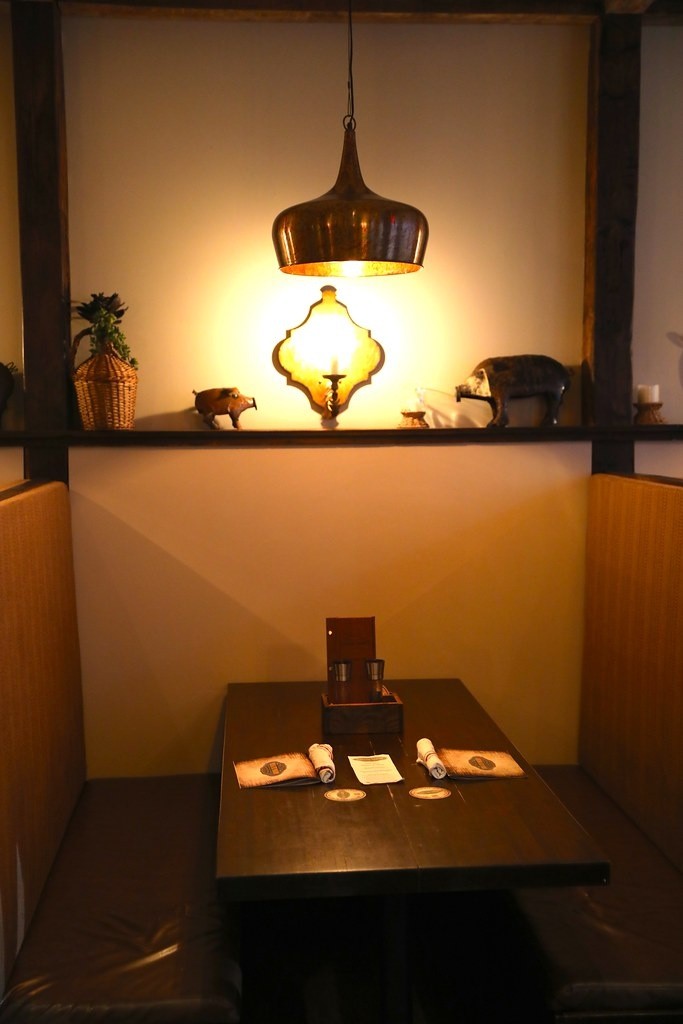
[73,292,139,431]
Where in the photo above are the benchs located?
[0,482,243,1024]
[498,471,683,1024]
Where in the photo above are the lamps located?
[278,283,381,419]
[272,0,429,278]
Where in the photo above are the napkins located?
[310,743,335,782]
[417,738,447,779]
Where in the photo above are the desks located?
[216,679,613,1024]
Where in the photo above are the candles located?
[637,384,660,403]
